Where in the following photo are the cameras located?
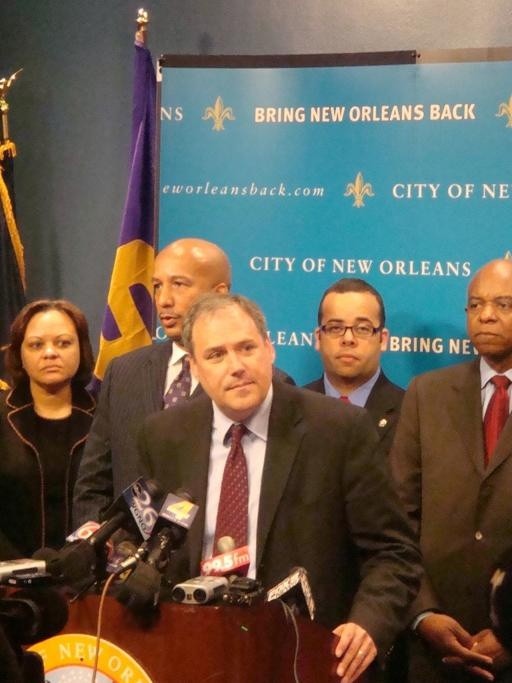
[0,585,69,663]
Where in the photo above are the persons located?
[299,277,406,457]
[0,299,96,563]
[137,294,424,682]
[393,258,512,681]
[71,238,294,559]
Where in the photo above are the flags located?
[0,135,27,392]
[90,34,158,405]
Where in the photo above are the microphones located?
[129,489,195,609]
[63,479,162,565]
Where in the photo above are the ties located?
[483,374,512,469]
[212,423,252,579]
[336,394,352,405]
[161,354,194,409]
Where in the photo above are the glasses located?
[317,323,383,339]
